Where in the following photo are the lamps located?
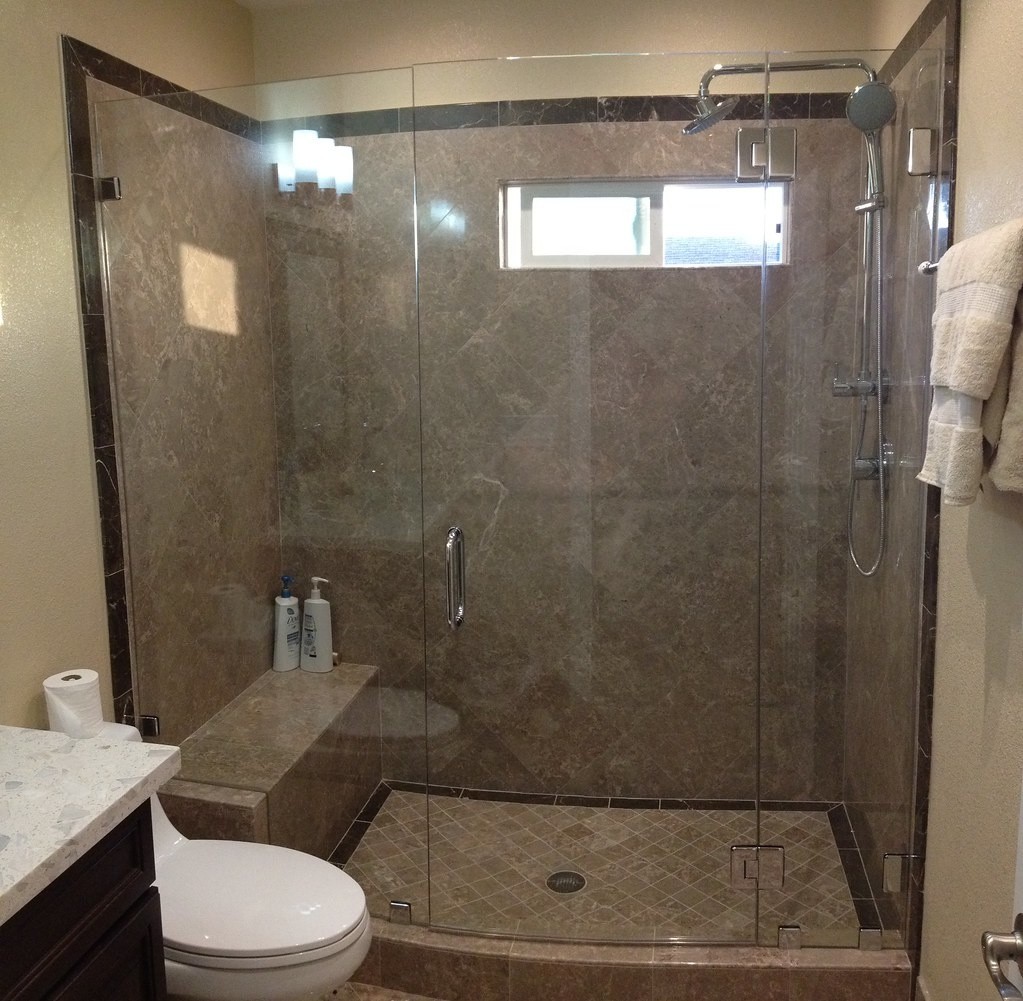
[271,128,352,210]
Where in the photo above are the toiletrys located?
[273,575,300,672]
[299,576,334,673]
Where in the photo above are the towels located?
[915,217,1023,507]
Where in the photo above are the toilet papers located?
[42,668,104,739]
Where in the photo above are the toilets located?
[92,720,373,1001]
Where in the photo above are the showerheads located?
[681,95,741,135]
[845,80,898,195]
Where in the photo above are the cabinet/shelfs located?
[0,797,167,1001]
